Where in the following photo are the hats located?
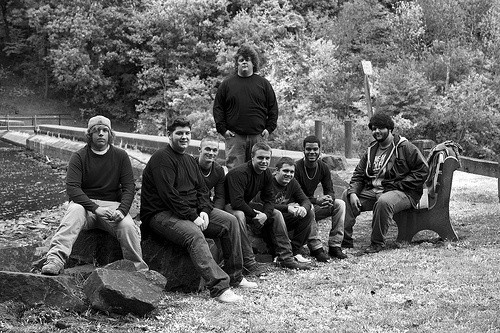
[88,115,111,129]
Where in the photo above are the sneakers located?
[42,259,64,275]
[310,247,331,261]
[217,288,241,302]
[328,245,348,259]
[235,277,258,288]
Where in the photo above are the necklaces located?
[304,163,318,180]
[200,169,211,178]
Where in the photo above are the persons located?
[223,142,310,276]
[195,137,264,276]
[253,157,313,255]
[293,135,347,262]
[140,117,258,302]
[212,44,278,169]
[341,111,429,254]
[41,115,149,275]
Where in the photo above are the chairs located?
[391,140,463,244]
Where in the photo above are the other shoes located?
[281,259,310,270]
[293,254,310,262]
[244,265,265,276]
[365,242,385,252]
